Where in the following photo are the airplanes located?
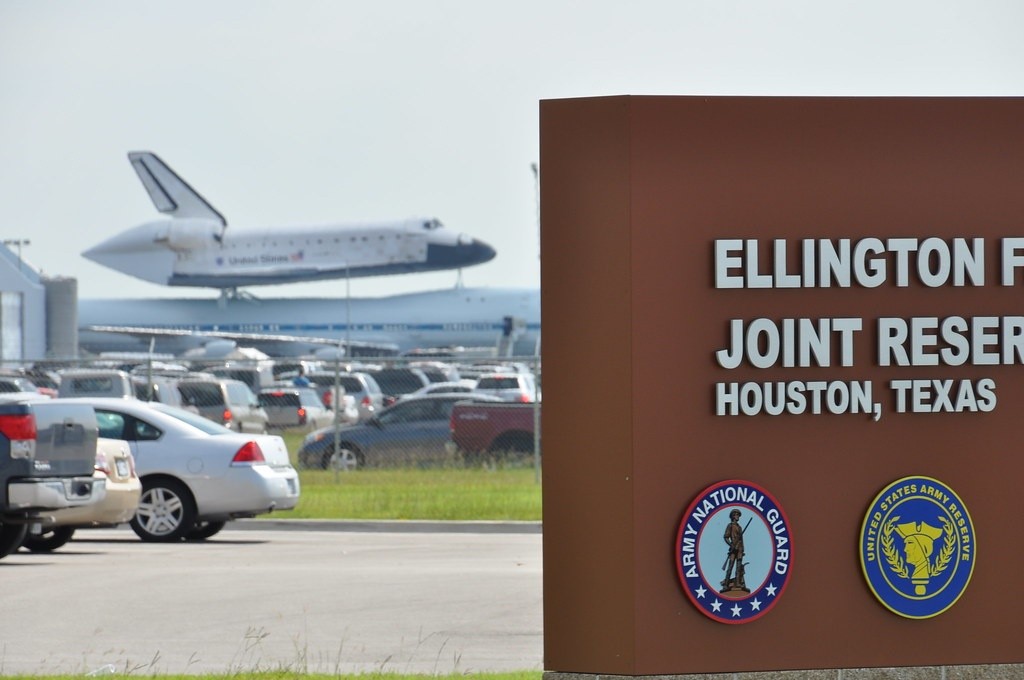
[75,151,541,363]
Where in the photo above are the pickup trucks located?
[444,401,539,470]
[0,403,100,561]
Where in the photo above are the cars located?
[0,360,532,436]
[257,388,335,430]
[300,394,506,473]
[472,372,536,402]
[399,380,474,398]
[24,438,142,554]
[42,397,302,543]
